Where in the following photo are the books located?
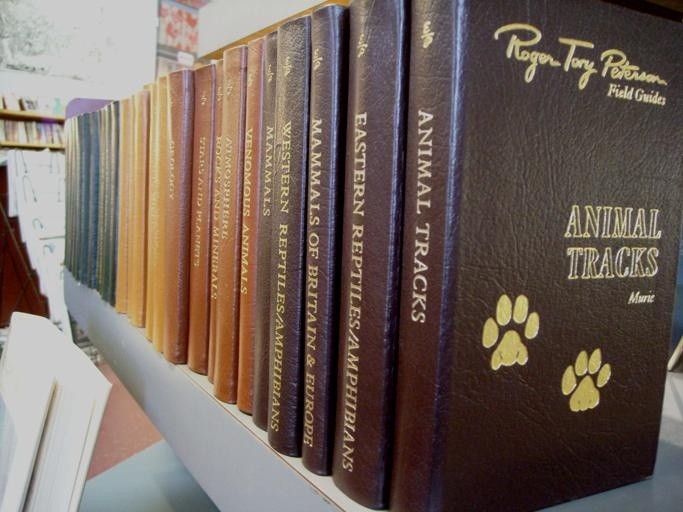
[0,118,63,146]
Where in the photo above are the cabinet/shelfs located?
[0,108,65,151]
